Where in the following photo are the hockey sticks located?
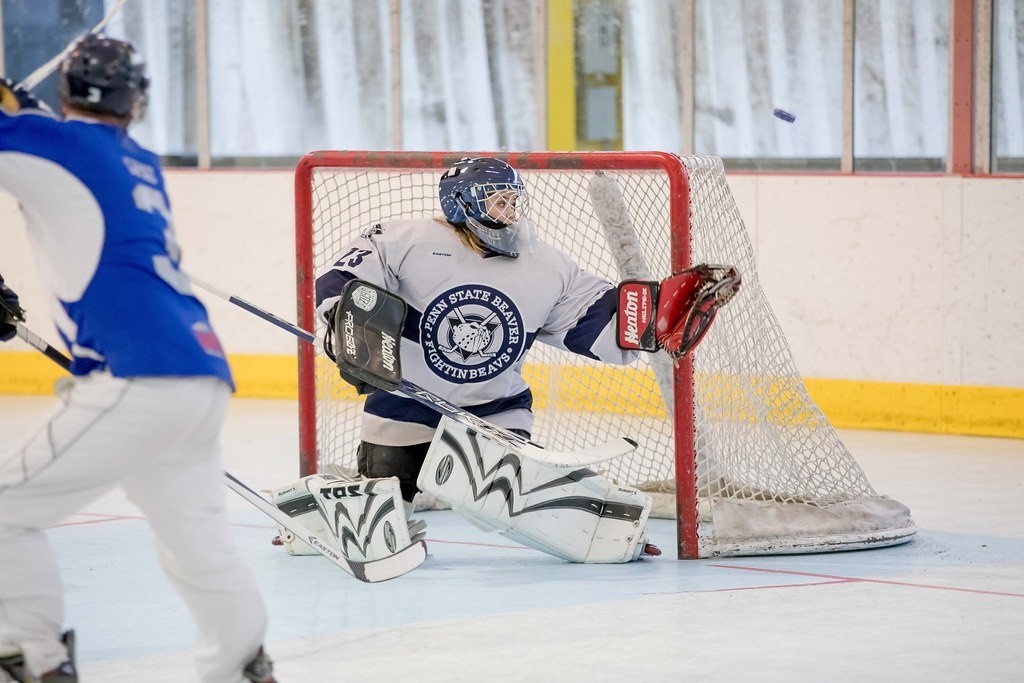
[9,318,426,586]
[187,260,636,469]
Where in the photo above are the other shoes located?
[242,647,278,683]
[0,630,77,683]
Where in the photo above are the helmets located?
[62,33,150,123]
[438,158,529,258]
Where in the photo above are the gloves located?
[0,275,26,341]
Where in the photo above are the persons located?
[0,33,278,683]
[304,155,743,565]
